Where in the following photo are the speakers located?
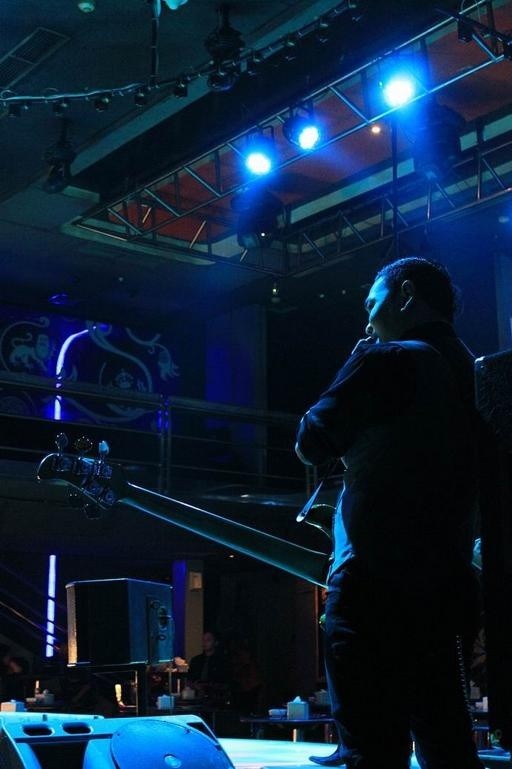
[65,578,175,668]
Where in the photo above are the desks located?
[240,715,338,743]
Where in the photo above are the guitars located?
[34,431,339,632]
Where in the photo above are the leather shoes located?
[310,748,345,766]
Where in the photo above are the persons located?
[295,259,485,769]
[187,632,228,684]
[308,454,356,766]
